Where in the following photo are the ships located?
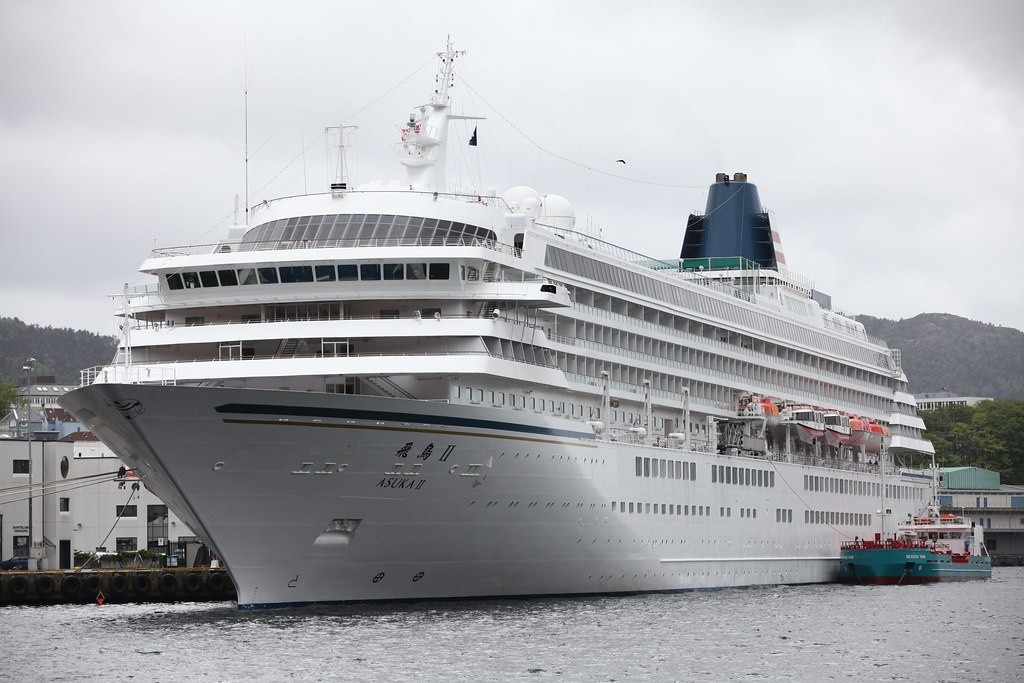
[56,33,942,610]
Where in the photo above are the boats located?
[839,455,992,585]
